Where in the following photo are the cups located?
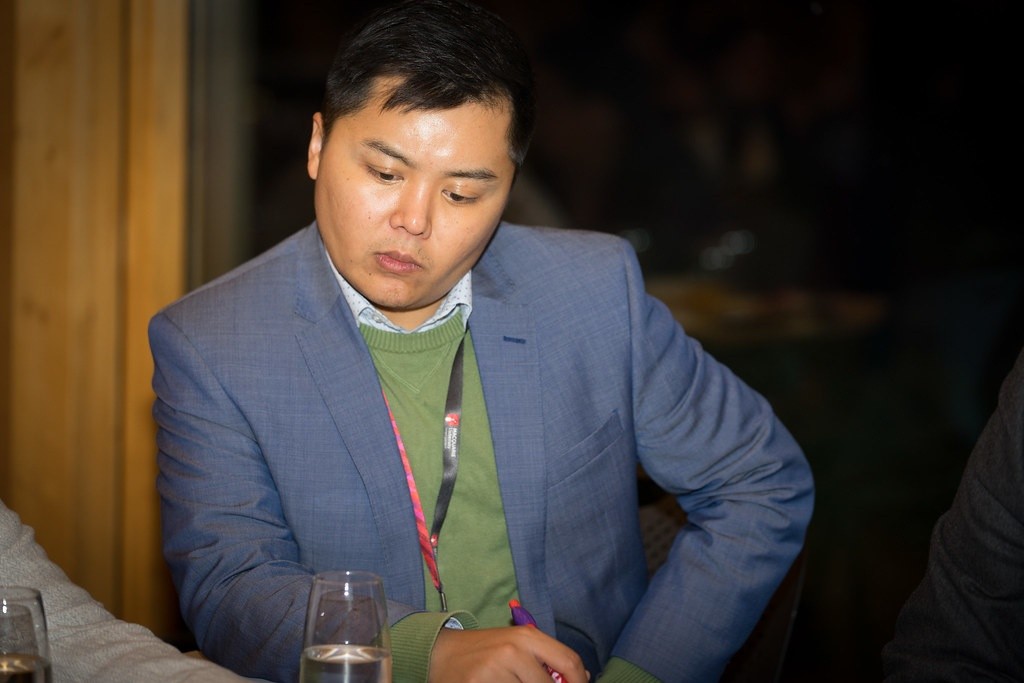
[299,570,392,683]
[0,585,52,683]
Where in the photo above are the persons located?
[878,348,1024,683]
[0,499,270,681]
[143,2,816,683]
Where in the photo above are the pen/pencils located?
[509,598,568,683]
